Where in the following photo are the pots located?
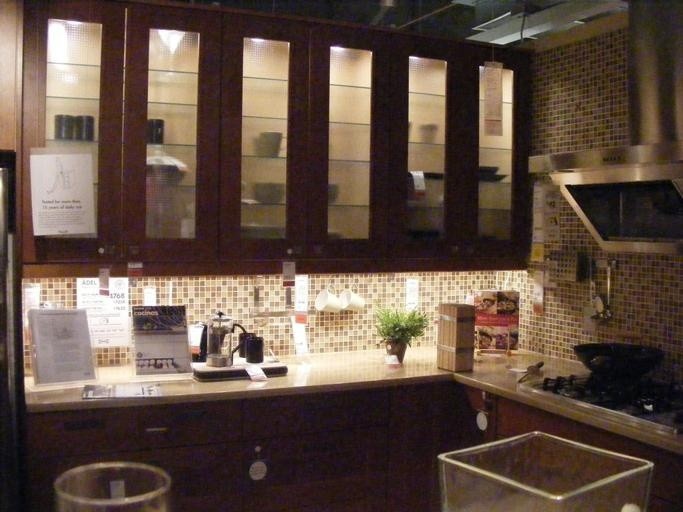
[573,343,665,385]
[146,150,188,184]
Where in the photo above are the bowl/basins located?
[254,182,285,203]
[479,166,499,174]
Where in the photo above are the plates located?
[480,174,510,183]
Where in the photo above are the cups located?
[339,284,365,311]
[55,115,94,141]
[418,124,438,143]
[253,131,287,157]
[200,310,246,367]
[147,119,164,144]
[239,333,264,363]
[314,284,341,313]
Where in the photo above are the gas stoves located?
[518,371,683,435]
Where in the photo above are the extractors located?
[528,0,683,255]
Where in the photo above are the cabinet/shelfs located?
[16,0,219,276]
[391,29,535,271]
[21,380,452,511]
[219,7,392,274]
[453,381,682,512]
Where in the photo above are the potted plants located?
[375,309,427,365]
